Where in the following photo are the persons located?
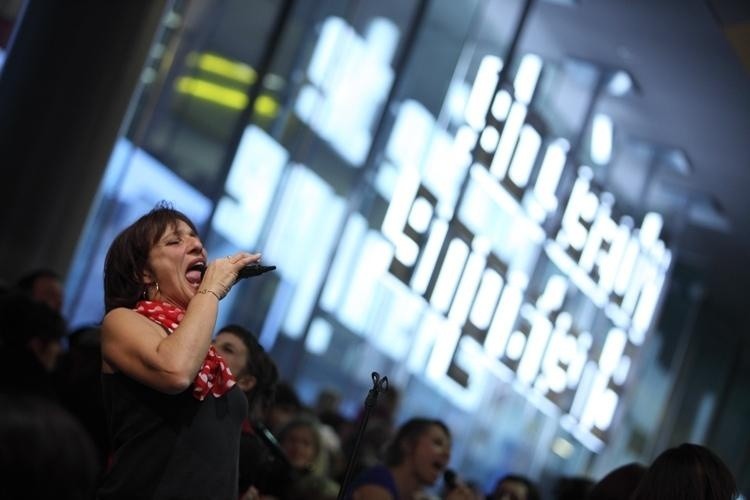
[480,440,739,500]
[212,325,475,499]
[1,267,107,499]
[91,203,279,498]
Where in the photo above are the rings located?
[222,255,232,259]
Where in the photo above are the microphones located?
[199,265,277,281]
